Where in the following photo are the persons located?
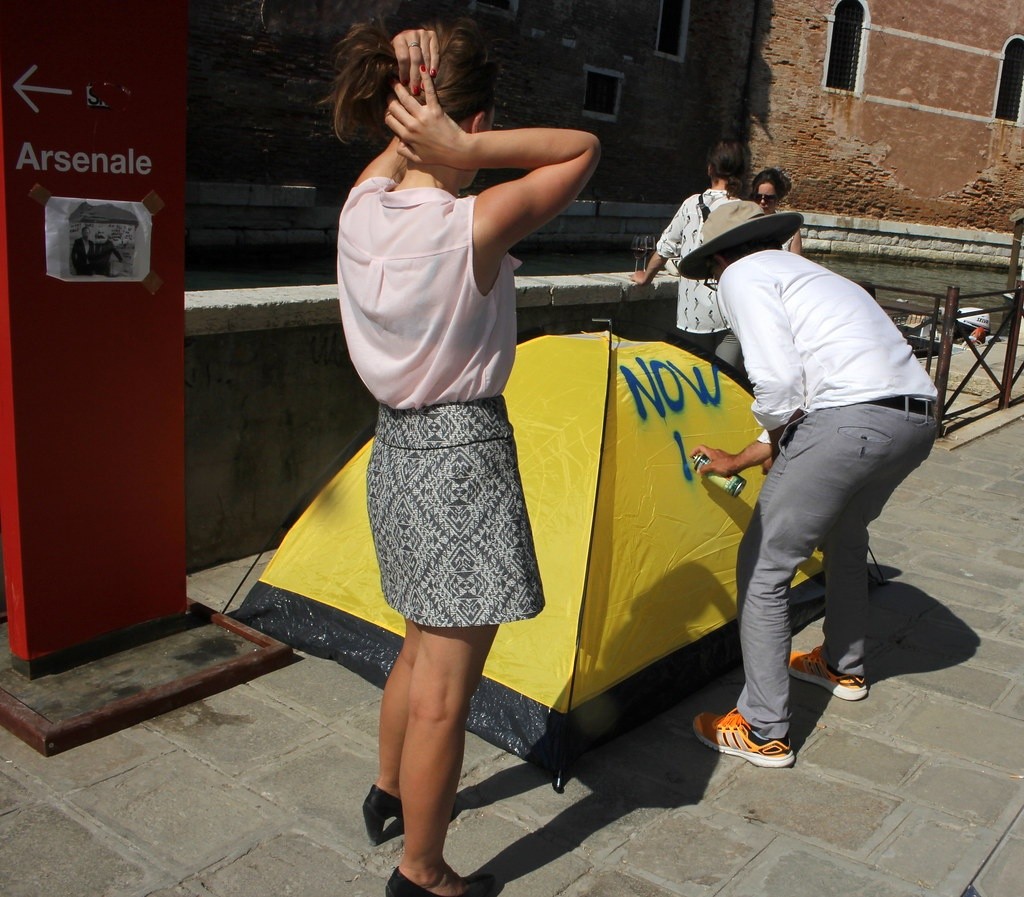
[678,201,937,769]
[71,227,127,276]
[319,14,601,897]
[628,138,801,379]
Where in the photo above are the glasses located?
[753,192,778,206]
[703,260,719,292]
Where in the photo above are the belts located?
[866,393,938,419]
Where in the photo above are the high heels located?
[360,784,405,846]
[382,867,497,897]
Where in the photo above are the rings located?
[408,41,420,49]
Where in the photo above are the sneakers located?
[691,711,797,769]
[786,647,870,702]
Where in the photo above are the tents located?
[220,318,888,792]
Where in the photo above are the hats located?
[676,200,805,279]
